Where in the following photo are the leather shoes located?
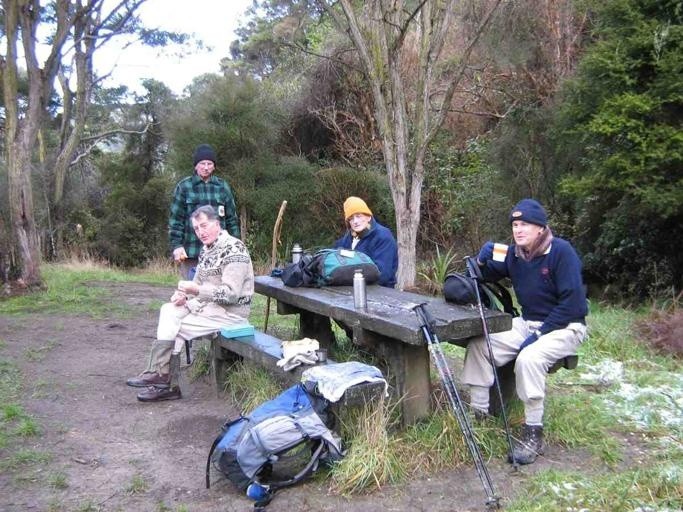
[125,373,172,388]
[137,385,182,402]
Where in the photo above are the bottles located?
[290,242,302,263]
[352,268,367,308]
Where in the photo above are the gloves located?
[519,332,538,350]
[478,241,493,264]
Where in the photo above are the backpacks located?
[298,247,380,288]
[478,279,519,318]
[209,381,342,502]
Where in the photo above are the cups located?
[313,348,327,362]
[490,242,508,262]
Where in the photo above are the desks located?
[252,273,512,432]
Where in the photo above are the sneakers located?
[509,424,545,465]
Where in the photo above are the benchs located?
[447,334,579,421]
[200,326,387,453]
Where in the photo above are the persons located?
[167,143,241,281]
[327,195,398,358]
[126,205,255,401]
[459,199,587,464]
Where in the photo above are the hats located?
[343,196,373,220]
[444,273,475,304]
[193,146,215,168]
[509,199,548,228]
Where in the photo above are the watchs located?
[534,330,542,338]
